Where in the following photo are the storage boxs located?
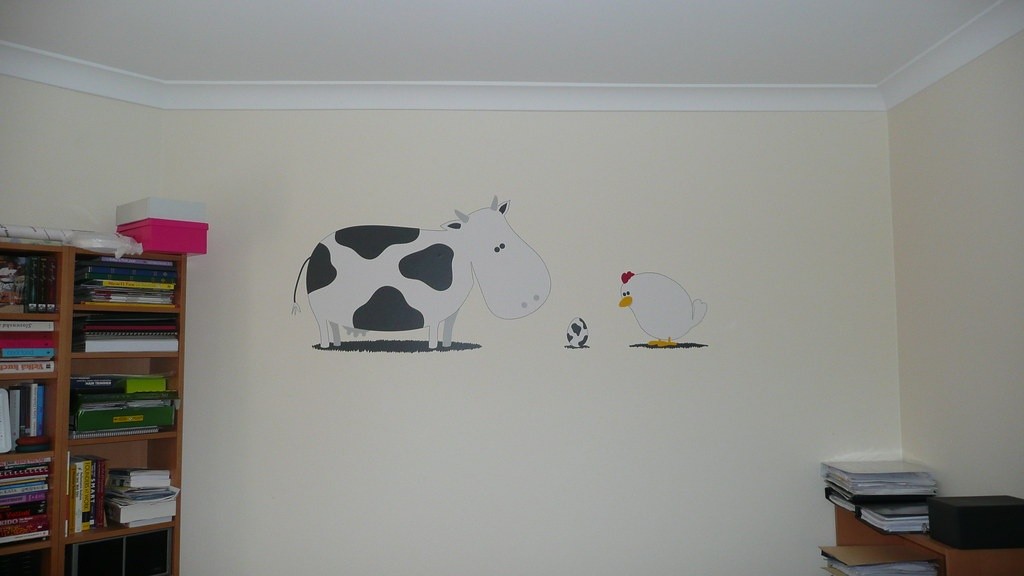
[117,198,209,256]
[928,495,1024,549]
[113,378,166,393]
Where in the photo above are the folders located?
[824,487,859,512]
[820,458,938,501]
[854,500,931,536]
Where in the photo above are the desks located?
[833,504,1024,576]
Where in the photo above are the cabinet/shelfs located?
[0,243,186,576]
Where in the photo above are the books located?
[0,248,180,547]
